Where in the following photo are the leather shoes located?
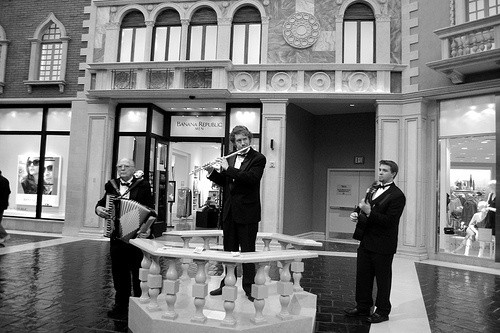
[367,312,389,323]
[343,306,371,317]
[210,287,222,295]
[245,288,254,302]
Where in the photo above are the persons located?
[343,160,406,324]
[448,180,497,256]
[21,157,54,195]
[95,157,151,319]
[0,170,12,242]
[202,125,267,301]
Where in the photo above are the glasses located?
[117,164,135,170]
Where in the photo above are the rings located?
[98,212,100,215]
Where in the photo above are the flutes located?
[189,145,252,175]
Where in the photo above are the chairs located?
[196,208,220,228]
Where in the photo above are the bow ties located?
[236,153,246,157]
[378,184,392,189]
[121,182,130,188]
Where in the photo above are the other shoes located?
[0,232,11,243]
[107,310,129,321]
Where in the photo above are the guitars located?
[353,187,375,241]
